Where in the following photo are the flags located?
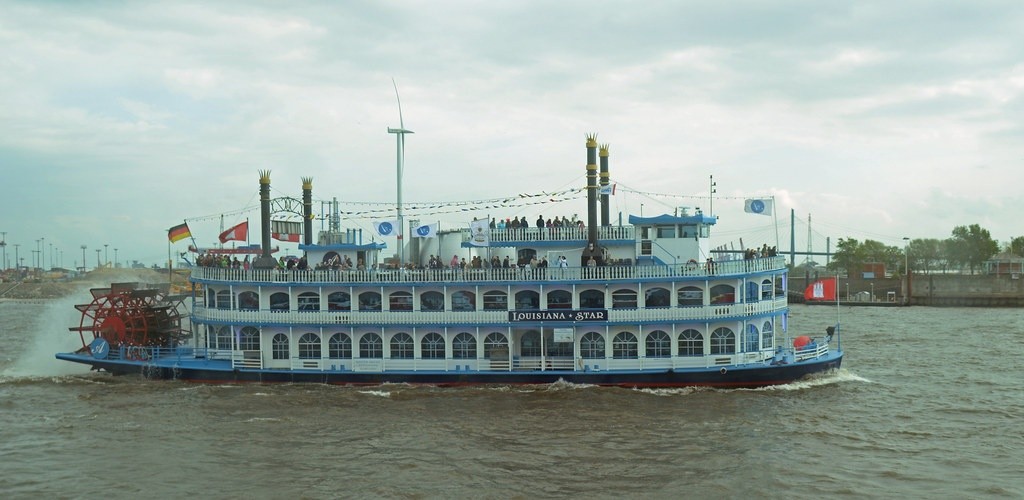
[412,222,437,238]
[168,223,191,244]
[469,218,489,246]
[371,219,402,238]
[804,278,837,301]
[599,184,616,195]
[219,221,247,244]
[744,199,772,216]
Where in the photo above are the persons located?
[705,259,717,275]
[586,255,624,279]
[535,214,587,240]
[276,253,377,282]
[489,216,529,242]
[426,253,568,280]
[382,261,425,282]
[744,244,777,272]
[196,253,251,281]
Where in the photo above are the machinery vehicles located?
[168,269,204,297]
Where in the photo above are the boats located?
[55,77,844,388]
